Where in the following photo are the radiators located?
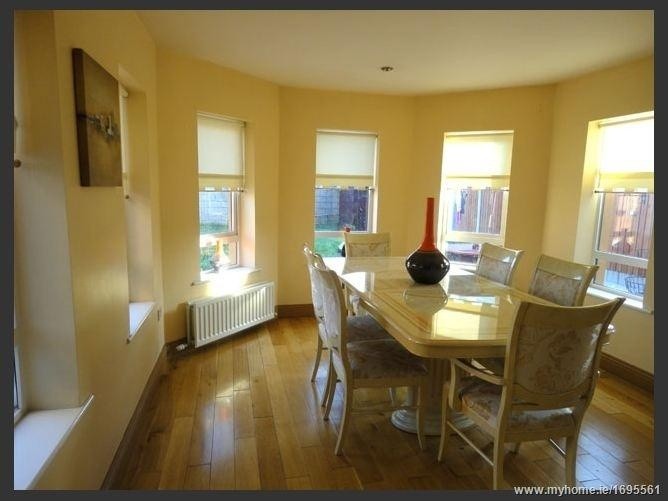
[180,280,281,351]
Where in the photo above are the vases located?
[337,226,350,256]
[210,238,229,273]
[405,196,452,287]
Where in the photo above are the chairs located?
[342,228,392,258]
[315,258,431,462]
[474,247,600,376]
[301,237,403,415]
[472,241,525,288]
[437,294,631,493]
